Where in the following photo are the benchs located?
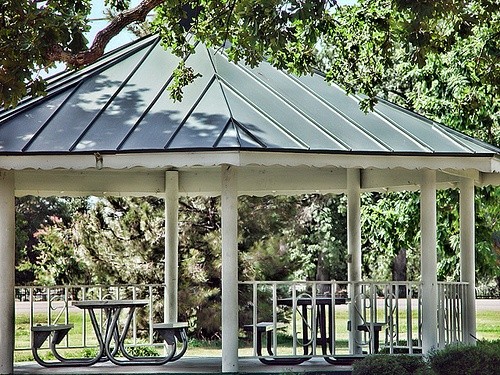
[347,322,383,363]
[246,322,286,364]
[30,324,73,366]
[148,319,191,366]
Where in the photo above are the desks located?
[275,295,346,363]
[73,300,147,364]
